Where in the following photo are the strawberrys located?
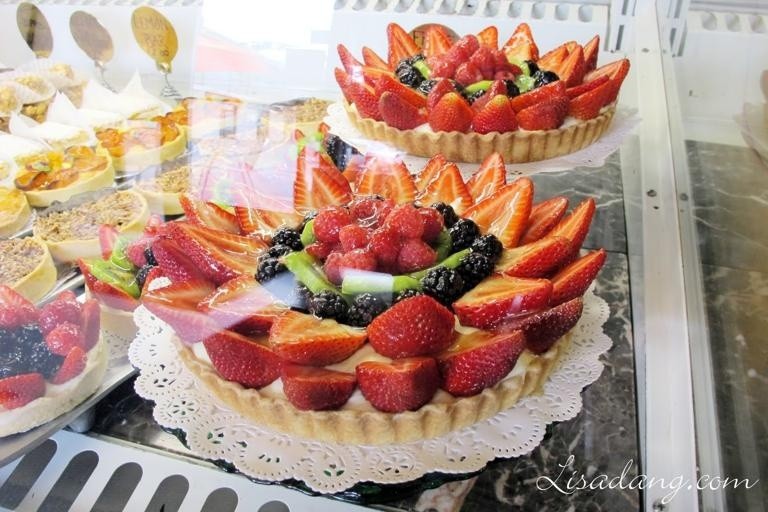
[335,22,630,135]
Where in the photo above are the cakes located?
[135,143,608,449]
[169,312,573,448]
[0,91,330,443]
[332,21,632,167]
